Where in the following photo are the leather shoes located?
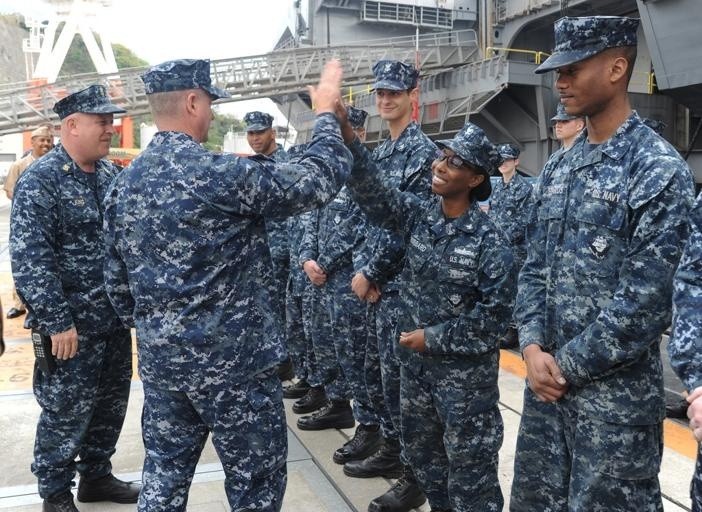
[368,475,427,512]
[282,380,310,399]
[343,442,404,479]
[6,307,26,320]
[292,387,327,414]
[296,399,356,431]
[77,472,144,505]
[42,491,79,512]
[332,424,385,464]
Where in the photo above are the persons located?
[8,85,144,512]
[4,126,52,318]
[508,13,701,512]
[101,55,533,512]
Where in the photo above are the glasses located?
[435,149,477,173]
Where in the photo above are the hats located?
[549,100,580,122]
[139,57,232,101]
[369,60,420,93]
[344,104,368,129]
[434,120,505,175]
[242,111,274,133]
[32,125,52,138]
[52,84,129,121]
[535,12,642,75]
[497,142,521,161]
[641,118,670,136]
